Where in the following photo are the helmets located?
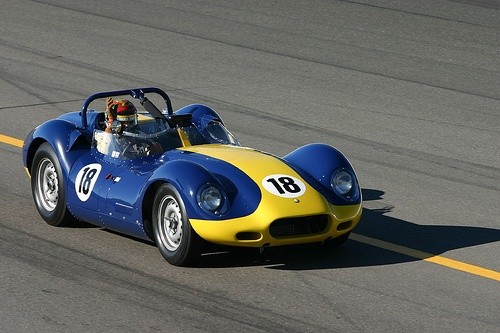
[115,98,138,130]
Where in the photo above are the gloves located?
[105,96,118,128]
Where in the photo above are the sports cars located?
[22,87,363,266]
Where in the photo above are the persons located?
[95,97,165,160]
